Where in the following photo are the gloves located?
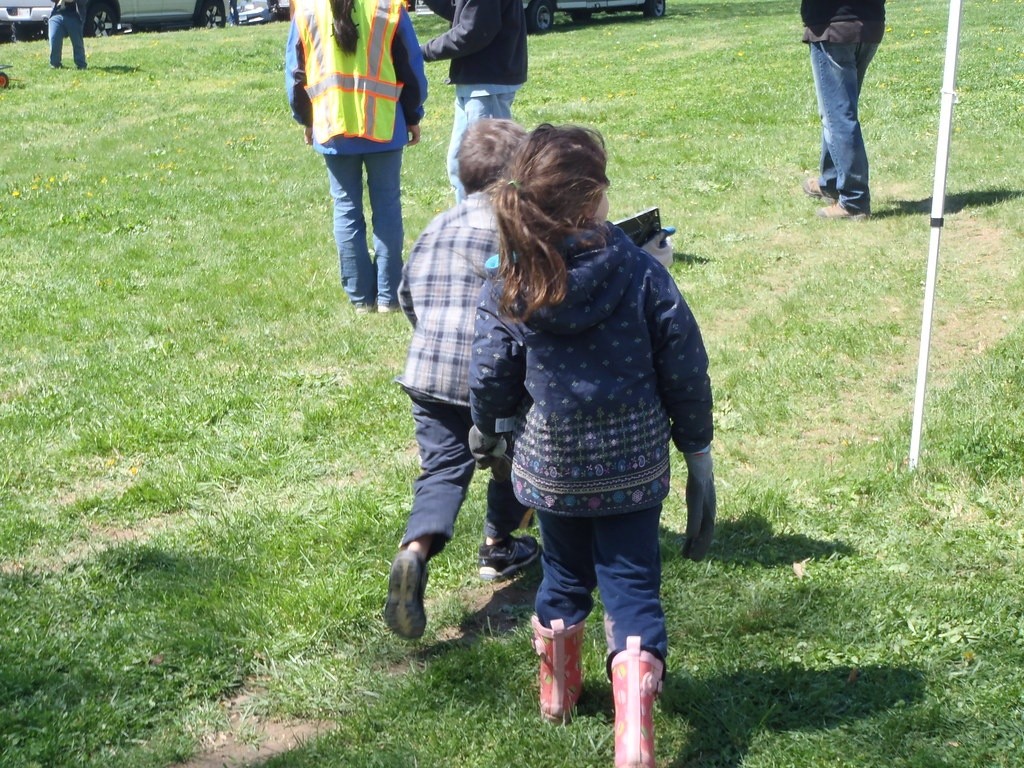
[468,424,507,469]
[682,447,716,561]
[639,227,675,271]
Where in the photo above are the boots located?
[611,636,663,768]
[530,614,585,724]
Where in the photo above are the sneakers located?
[479,536,539,579]
[383,550,429,640]
[804,177,832,201]
[815,202,869,221]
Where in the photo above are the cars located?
[518,1,665,36]
[1,0,293,44]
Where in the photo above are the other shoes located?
[376,306,400,312]
[355,306,375,316]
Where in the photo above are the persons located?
[47,0,86,70]
[421,0,527,204]
[382,118,717,768]
[227,0,238,27]
[800,0,885,218]
[285,0,430,312]
